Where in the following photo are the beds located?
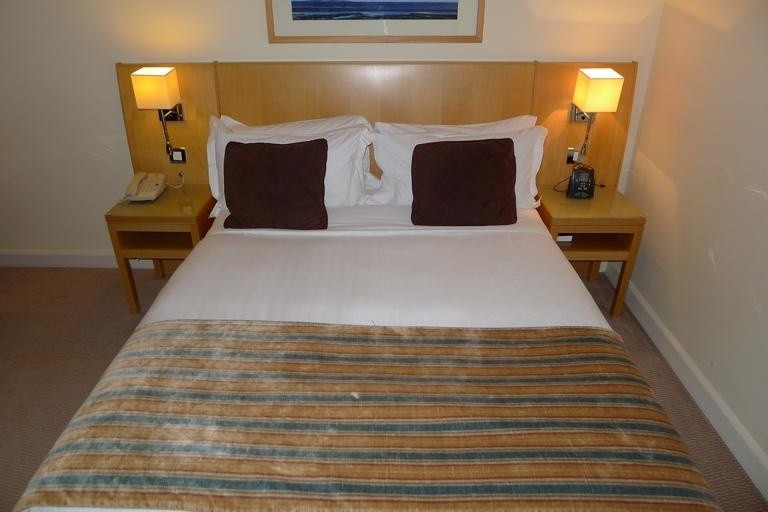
[13,61,724,512]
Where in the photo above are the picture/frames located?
[264,0,486,43]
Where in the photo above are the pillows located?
[219,113,369,133]
[206,115,371,217]
[352,124,550,209]
[411,137,517,226]
[224,137,330,230]
[375,114,539,136]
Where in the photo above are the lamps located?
[568,67,622,164]
[131,67,188,162]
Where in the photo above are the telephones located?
[125,172,167,202]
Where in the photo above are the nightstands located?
[105,185,213,316]
[535,183,645,319]
